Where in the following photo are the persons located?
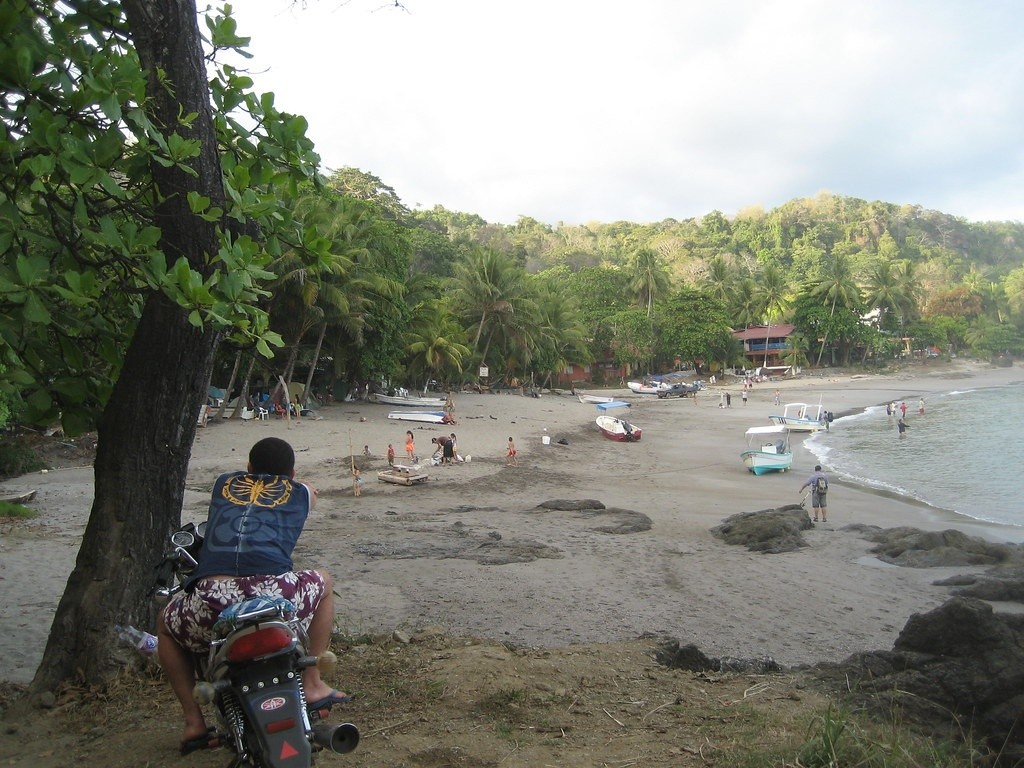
[432,434,456,467]
[619,377,624,388]
[776,390,781,405]
[571,381,575,395]
[603,376,608,388]
[799,465,828,522]
[312,378,372,401]
[405,430,415,458]
[742,390,747,404]
[151,438,347,746]
[900,402,909,418]
[898,419,910,435]
[887,403,892,414]
[363,445,371,455]
[246,391,301,420]
[726,392,731,408]
[823,410,829,431]
[504,437,517,467]
[918,398,925,413]
[388,444,392,466]
[351,464,361,496]
[743,374,753,388]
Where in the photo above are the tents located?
[275,383,321,409]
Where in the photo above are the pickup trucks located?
[656,383,698,399]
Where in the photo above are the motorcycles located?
[144,518,361,768]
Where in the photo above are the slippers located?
[308,689,350,711]
[178,729,214,755]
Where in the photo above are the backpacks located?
[816,473,828,494]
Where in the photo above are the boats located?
[625,380,671,395]
[738,425,794,477]
[372,388,454,408]
[388,409,454,426]
[769,402,829,432]
[595,415,642,442]
[205,395,311,419]
[579,393,615,404]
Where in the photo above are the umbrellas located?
[204,385,223,399]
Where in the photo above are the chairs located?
[258,407,270,420]
[393,388,402,396]
[400,387,409,396]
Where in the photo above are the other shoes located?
[813,517,818,521]
[823,519,827,521]
